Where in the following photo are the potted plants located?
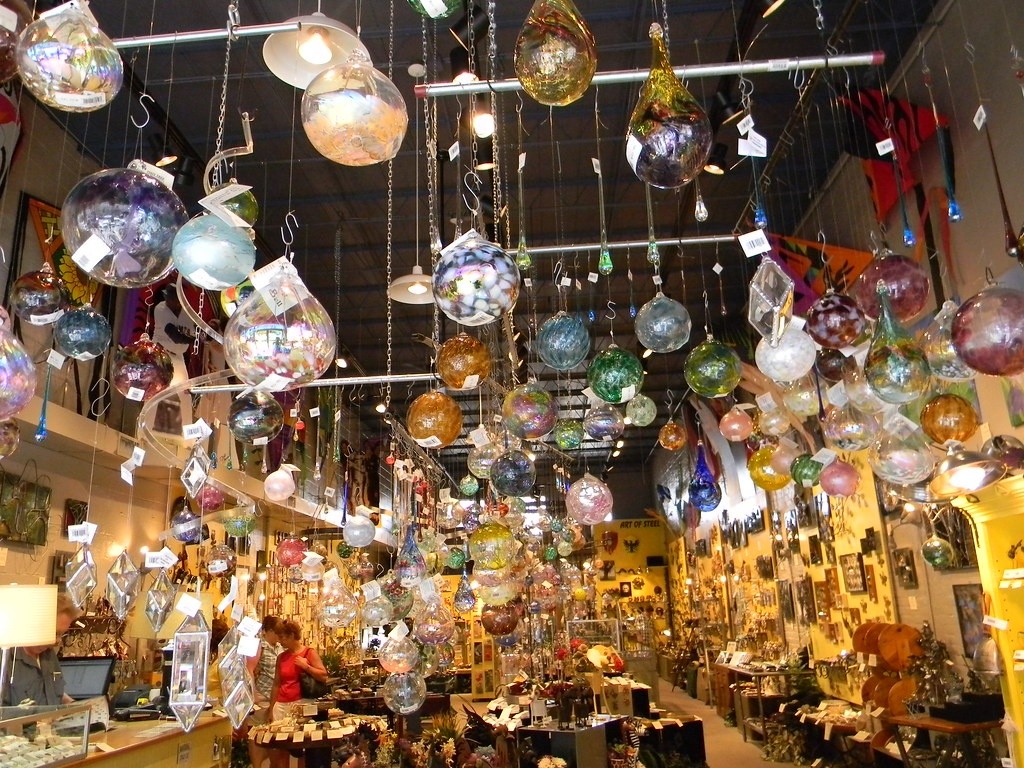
[252,717,358,768]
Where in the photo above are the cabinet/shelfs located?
[516,718,606,768]
[689,598,727,711]
[471,610,495,703]
[617,600,667,652]
[566,619,620,654]
[659,648,691,683]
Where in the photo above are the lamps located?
[460,103,497,139]
[450,7,491,51]
[714,89,741,124]
[449,47,483,82]
[930,445,1007,496]
[376,400,387,413]
[335,353,350,367]
[585,558,593,568]
[263,1,372,90]
[387,63,437,304]
[472,134,495,170]
[703,142,729,175]
[478,196,507,218]
[886,477,956,504]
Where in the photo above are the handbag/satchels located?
[300,671,331,699]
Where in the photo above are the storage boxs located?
[277,389,392,532]
[628,719,660,765]
[660,715,705,763]
[629,672,659,703]
[613,677,633,717]
[620,640,656,672]
[604,676,619,714]
[687,664,704,698]
[698,667,712,701]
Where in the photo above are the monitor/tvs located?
[57,656,116,701]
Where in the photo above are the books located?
[52,695,117,733]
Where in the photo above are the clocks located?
[233,565,250,608]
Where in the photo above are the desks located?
[728,666,814,748]
[711,661,732,719]
[889,713,1004,768]
[794,710,867,766]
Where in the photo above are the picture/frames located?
[952,584,985,658]
[839,552,867,590]
[731,519,740,551]
[924,504,977,572]
[815,582,831,622]
[241,532,252,557]
[600,561,616,581]
[784,510,801,550]
[619,582,632,596]
[808,535,822,564]
[775,580,793,618]
[872,469,903,515]
[796,577,816,622]
[891,548,918,586]
[269,531,359,652]
[737,517,748,547]
[2,191,124,427]
[825,568,839,607]
[64,498,89,535]
[745,506,765,534]
[815,492,835,542]
[225,532,238,554]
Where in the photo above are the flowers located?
[412,713,471,768]
[542,680,574,699]
[574,679,596,703]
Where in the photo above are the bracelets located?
[305,665,310,672]
[71,700,76,702]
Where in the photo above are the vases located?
[553,700,575,730]
[575,702,593,728]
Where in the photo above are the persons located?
[247,615,285,768]
[267,619,327,768]
[634,605,664,676]
[0,592,79,729]
[206,618,231,702]
